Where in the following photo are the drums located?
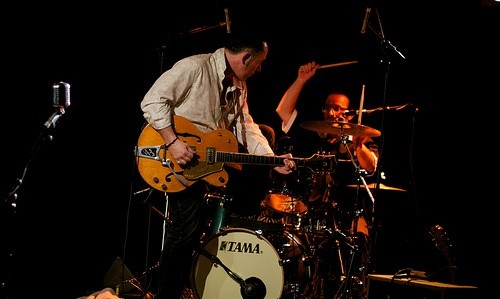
[190,222,312,299]
[263,166,314,216]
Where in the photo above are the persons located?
[276,61,380,299]
[140,32,297,299]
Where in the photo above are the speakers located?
[103,256,142,293]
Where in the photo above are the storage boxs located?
[366,273,478,299]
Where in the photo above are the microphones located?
[43,82,71,129]
[361,3,373,33]
[245,284,254,296]
[224,7,231,33]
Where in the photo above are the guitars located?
[135,115,337,193]
[428,224,459,288]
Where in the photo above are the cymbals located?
[300,116,381,138]
[345,183,407,191]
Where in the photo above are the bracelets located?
[167,137,178,148]
